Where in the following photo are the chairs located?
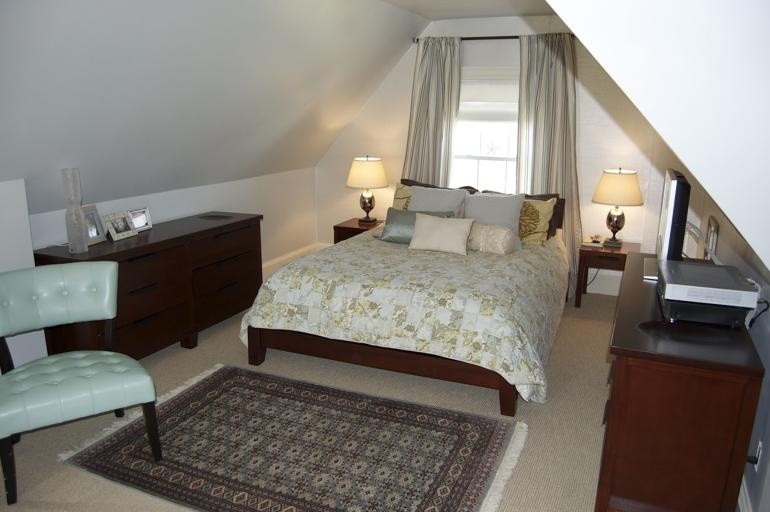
[0,261,163,505]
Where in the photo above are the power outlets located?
[753,441,763,472]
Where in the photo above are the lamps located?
[591,168,644,248]
[345,155,388,225]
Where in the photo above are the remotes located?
[581,242,604,249]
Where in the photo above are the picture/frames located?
[127,207,152,232]
[102,210,138,242]
[81,204,107,247]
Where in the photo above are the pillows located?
[381,179,564,256]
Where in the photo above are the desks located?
[594,251,765,512]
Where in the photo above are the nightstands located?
[574,239,641,308]
[333,218,385,244]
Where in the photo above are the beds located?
[236,179,571,418]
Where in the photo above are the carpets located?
[57,361,529,512]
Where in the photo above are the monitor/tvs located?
[643,168,691,281]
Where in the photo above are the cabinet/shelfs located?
[191,220,263,335]
[33,234,192,360]
